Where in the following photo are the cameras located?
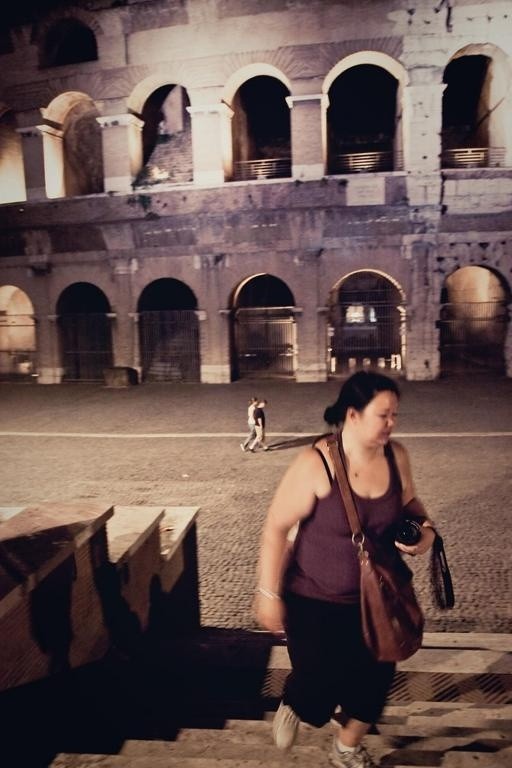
[395,515,424,547]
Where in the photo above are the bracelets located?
[256,586,284,602]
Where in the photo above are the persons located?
[252,365,437,766]
[246,398,273,452]
[240,396,259,453]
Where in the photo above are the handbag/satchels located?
[358,548,427,671]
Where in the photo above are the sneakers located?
[239,442,272,453]
[326,737,381,768]
[271,696,301,752]
